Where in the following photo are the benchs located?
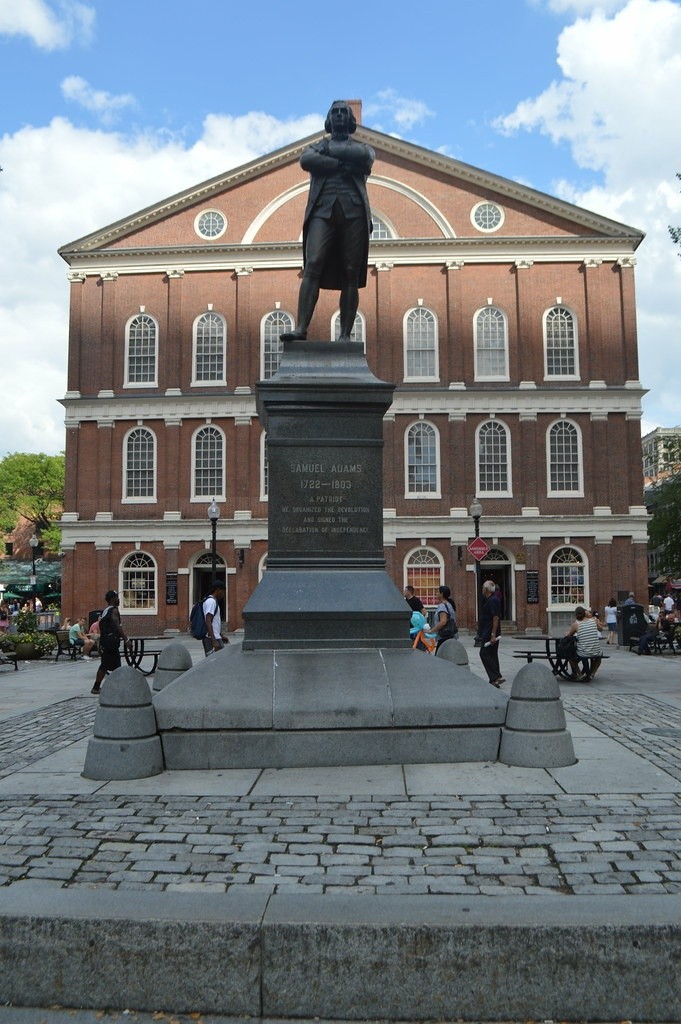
[512,650,611,683]
[628,622,681,656]
[0,638,19,671]
[54,629,84,661]
[119,650,163,676]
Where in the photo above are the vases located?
[2,643,46,661]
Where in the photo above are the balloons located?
[409,611,437,652]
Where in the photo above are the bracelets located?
[491,633,495,636]
[430,630,432,632]
[126,639,129,643]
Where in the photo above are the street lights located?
[29,534,39,591]
[469,493,483,648]
[207,496,220,587]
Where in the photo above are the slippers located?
[492,679,506,686]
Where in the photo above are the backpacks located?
[0,606,8,621]
[190,597,218,640]
[100,605,121,650]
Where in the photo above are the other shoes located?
[589,674,595,680]
[575,673,586,681]
[632,649,641,656]
[81,655,90,660]
[642,652,652,655]
[607,641,611,645]
[91,688,101,694]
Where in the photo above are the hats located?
[594,611,600,618]
[212,580,227,590]
[105,591,121,600]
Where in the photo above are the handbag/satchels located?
[439,618,458,639]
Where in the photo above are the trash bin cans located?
[617,604,644,646]
[89,610,103,629]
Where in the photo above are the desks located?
[510,635,607,682]
[121,636,174,676]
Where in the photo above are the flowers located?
[0,609,59,657]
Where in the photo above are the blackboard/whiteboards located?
[527,570,538,603]
[165,571,177,606]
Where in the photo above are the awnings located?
[648,574,658,577]
[652,573,668,584]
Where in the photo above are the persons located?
[474,579,506,688]
[423,586,459,656]
[604,598,618,645]
[564,607,603,680]
[404,585,427,651]
[89,615,102,654]
[279,100,376,342]
[91,590,133,694]
[202,584,229,657]
[624,591,675,655]
[0,596,42,634]
[60,617,95,659]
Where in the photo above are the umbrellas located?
[45,591,63,604]
[4,591,24,604]
